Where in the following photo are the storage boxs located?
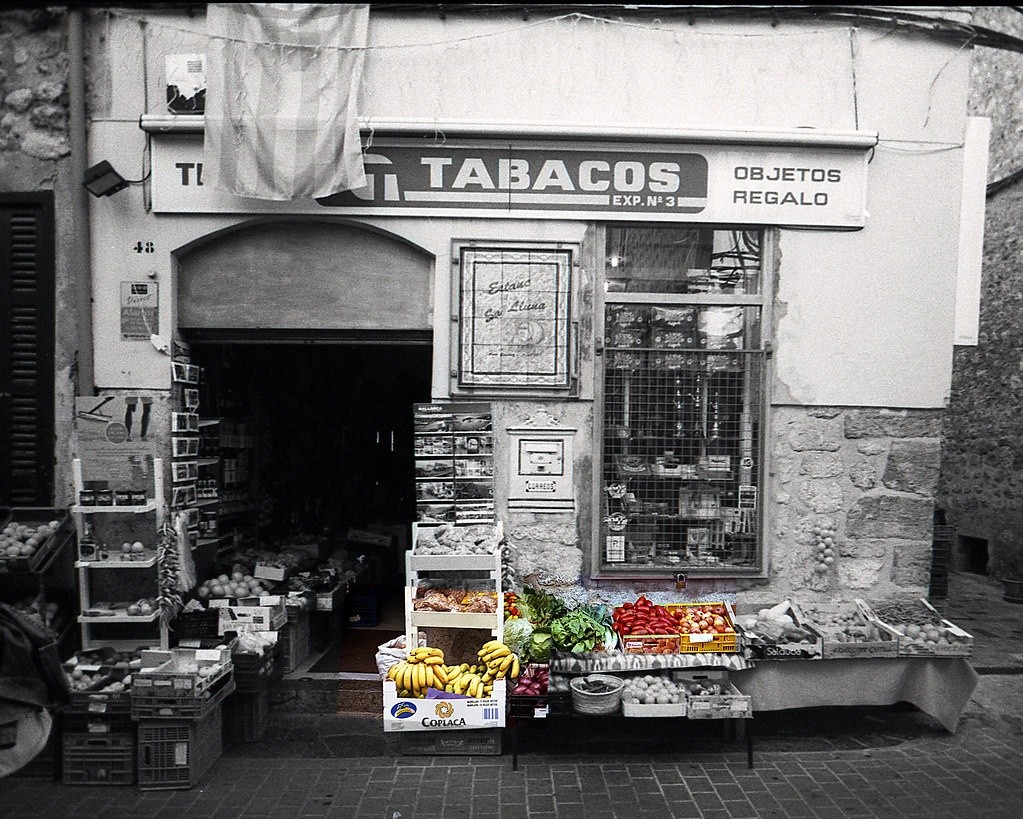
[403,521,507,656]
[60,635,281,793]
[508,586,975,725]
[381,668,504,756]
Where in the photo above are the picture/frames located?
[445,234,586,404]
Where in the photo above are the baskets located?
[570,673,624,714]
